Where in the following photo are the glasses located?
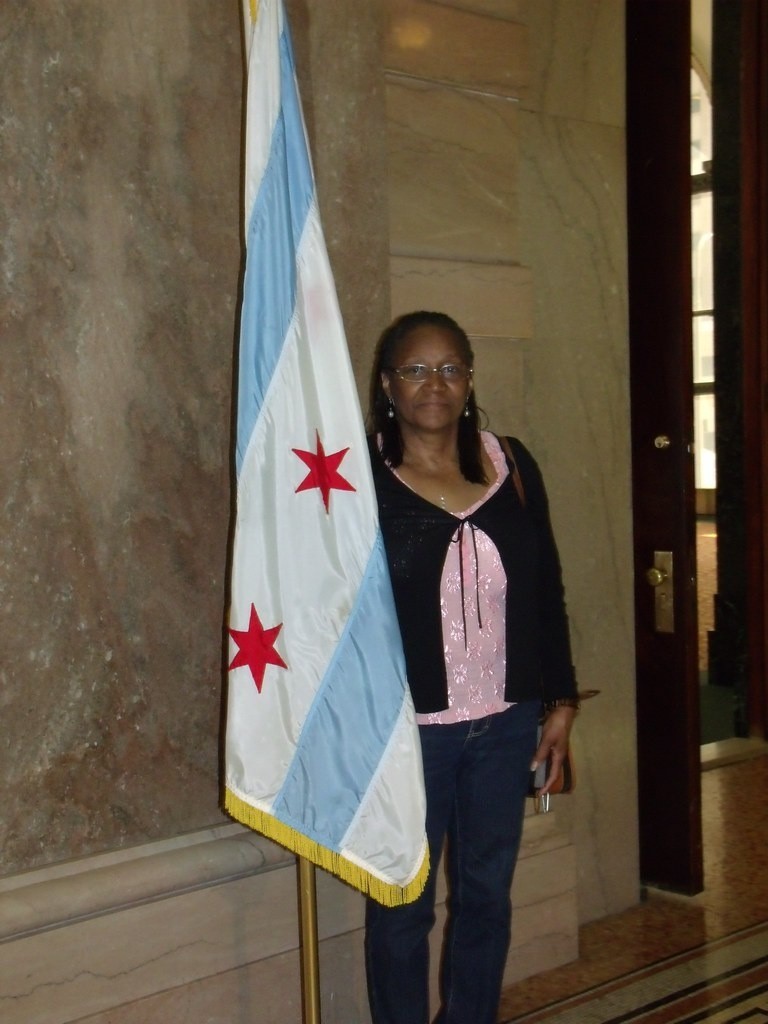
[383,363,471,383]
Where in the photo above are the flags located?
[216,0,430,906]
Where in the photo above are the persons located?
[360,309,580,1019]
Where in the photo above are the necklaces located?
[440,495,445,508]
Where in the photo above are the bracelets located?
[552,699,580,712]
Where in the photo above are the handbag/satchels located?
[525,706,577,797]
